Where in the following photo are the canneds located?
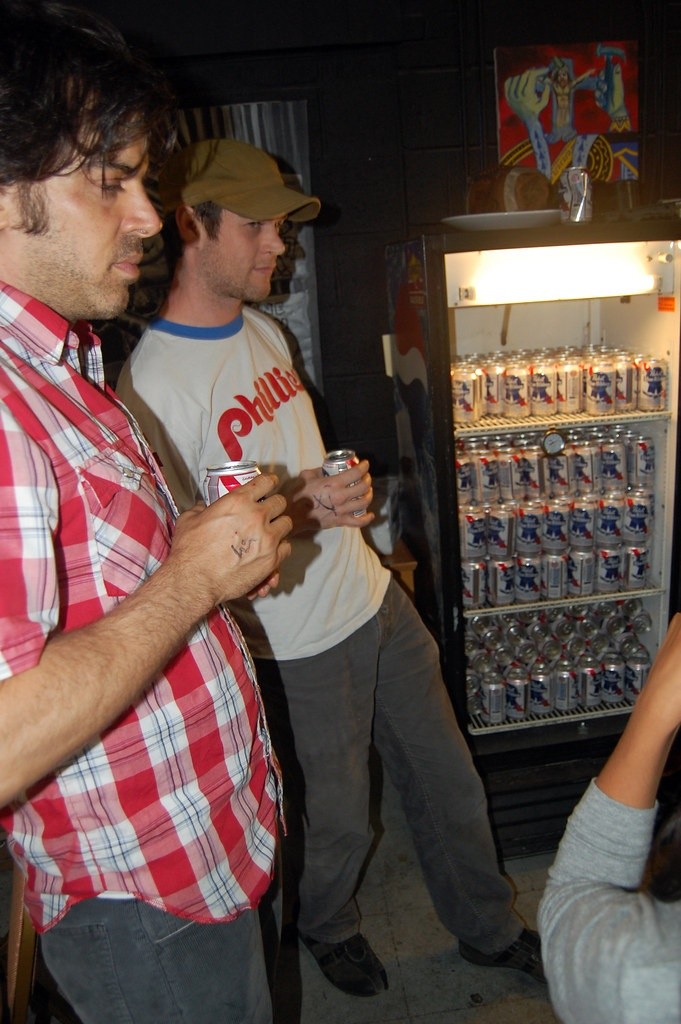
[204,460,262,509]
[450,343,666,423]
[322,449,367,518]
[466,598,655,726]
[456,427,657,607]
[557,165,591,224]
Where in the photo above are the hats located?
[156,136,321,222]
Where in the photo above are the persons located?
[112,135,549,996]
[0,0,293,1024]
[537,612,681,1024]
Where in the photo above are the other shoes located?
[297,926,389,996]
[458,928,549,989]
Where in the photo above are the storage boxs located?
[360,471,404,556]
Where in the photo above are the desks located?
[378,539,418,620]
